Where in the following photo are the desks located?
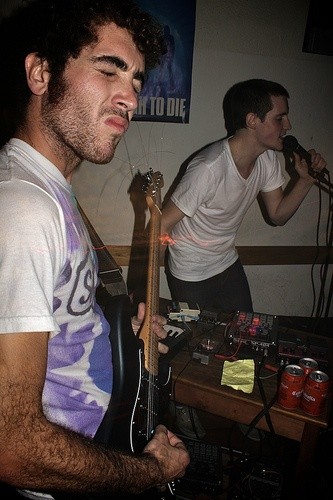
[156,298,333,500]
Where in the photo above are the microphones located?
[283,135,329,174]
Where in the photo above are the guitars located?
[90,165,182,500]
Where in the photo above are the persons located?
[0,0,193,500]
[158,77,327,444]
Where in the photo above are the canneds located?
[276,357,329,416]
